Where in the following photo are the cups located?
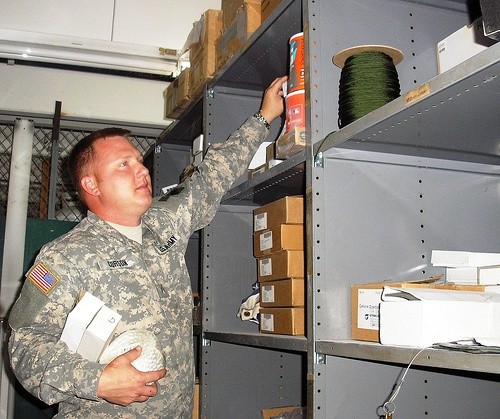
[286,30,306,94]
[284,89,306,132]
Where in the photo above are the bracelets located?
[253,112,273,130]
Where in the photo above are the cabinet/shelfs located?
[151,0,500,419]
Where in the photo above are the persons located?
[7,76,288,419]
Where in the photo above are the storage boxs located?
[252,195,305,336]
[163,0,282,122]
[436,25,486,74]
[349,250,500,346]
